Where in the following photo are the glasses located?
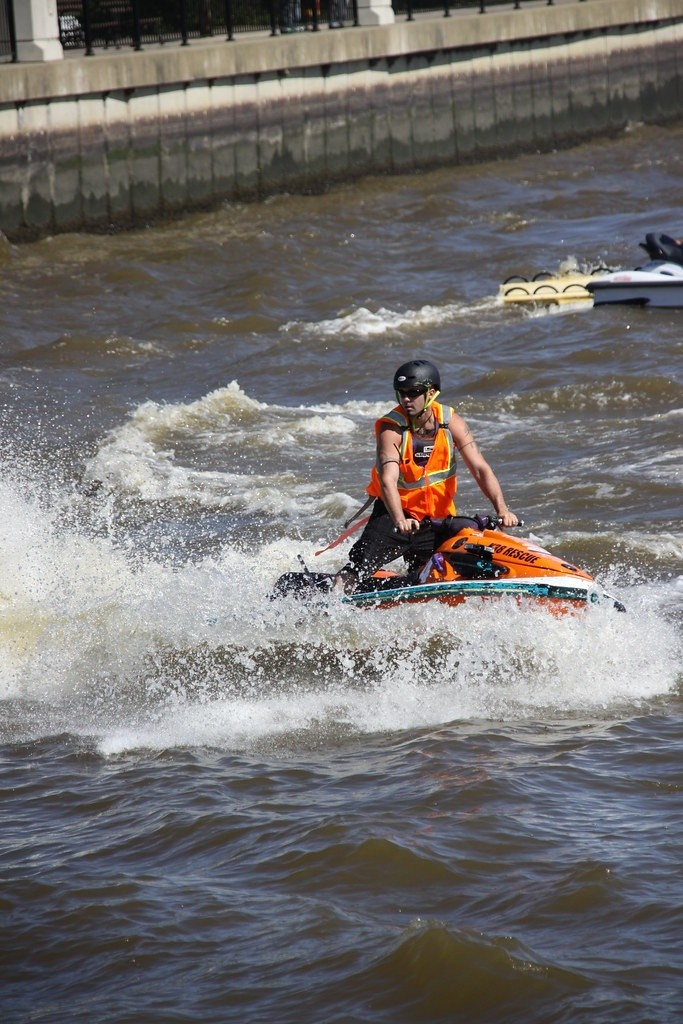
[398,388,427,398]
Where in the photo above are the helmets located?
[393,360,440,391]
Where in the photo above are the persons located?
[331,358,519,594]
